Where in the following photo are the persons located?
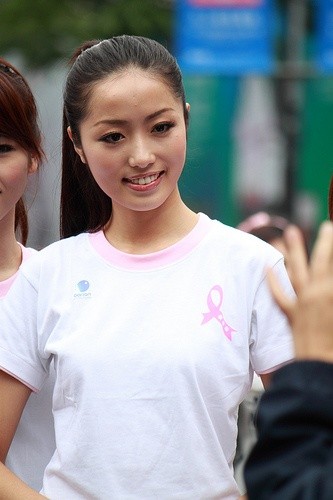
[0,34,295,498]
[0,57,53,498]
[243,220,333,500]
